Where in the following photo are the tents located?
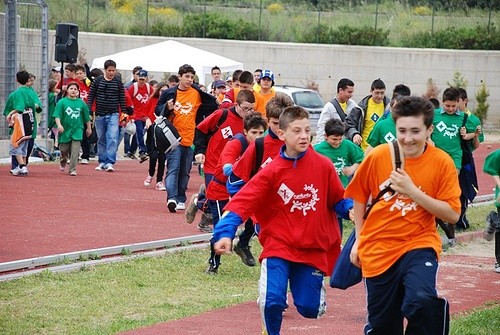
[90,40,245,88]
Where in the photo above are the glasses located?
[239,104,254,112]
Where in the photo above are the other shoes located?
[70,170,77,176]
[485,214,494,240]
[494,262,500,272]
[105,163,113,172]
[60,166,65,170]
[81,159,88,163]
[95,163,105,171]
[123,151,149,164]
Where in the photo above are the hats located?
[138,70,148,79]
[52,67,63,73]
[212,81,227,88]
[260,69,274,86]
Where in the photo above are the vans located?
[272,85,324,140]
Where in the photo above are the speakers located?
[55,23,79,63]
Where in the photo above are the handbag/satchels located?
[124,119,136,135]
[459,113,473,150]
[329,230,362,290]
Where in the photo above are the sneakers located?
[205,258,219,274]
[167,200,178,213]
[10,166,20,175]
[156,182,166,191]
[177,202,186,210]
[18,166,28,174]
[185,193,198,223]
[144,173,153,186]
[234,244,255,266]
[197,222,213,232]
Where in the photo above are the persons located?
[186,61,484,276]
[3,59,181,191]
[344,95,462,335]
[213,107,371,335]
[483,149,500,273]
[154,63,220,214]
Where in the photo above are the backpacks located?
[145,102,182,159]
